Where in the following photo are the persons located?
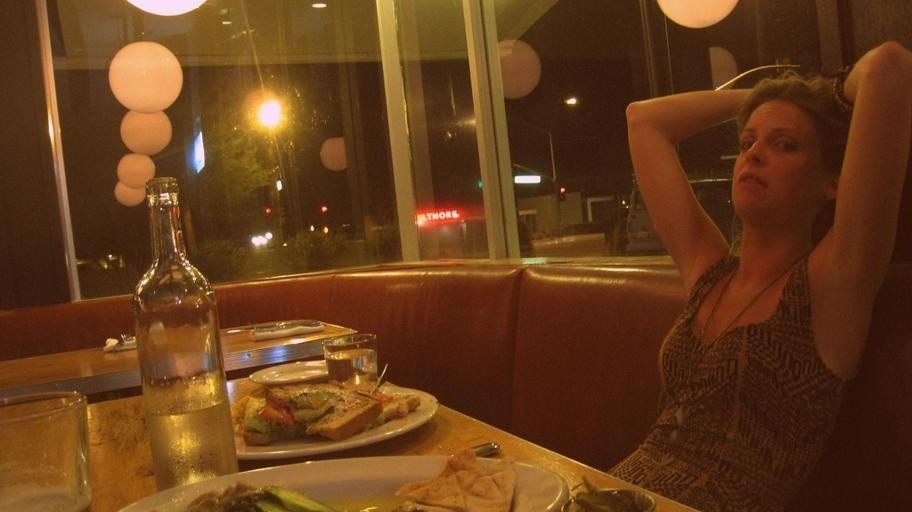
[610,38,912,511]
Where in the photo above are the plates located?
[114,454,570,512]
[229,379,440,463]
[247,360,330,385]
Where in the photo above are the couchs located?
[1,261,910,512]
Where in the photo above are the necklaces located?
[686,250,811,389]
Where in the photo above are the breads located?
[395,449,517,512]
[244,381,421,445]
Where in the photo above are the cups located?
[0,385,95,512]
[321,331,380,389]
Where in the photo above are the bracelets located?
[830,61,853,113]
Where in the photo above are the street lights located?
[547,95,578,227]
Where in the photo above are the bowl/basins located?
[559,487,656,512]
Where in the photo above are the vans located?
[625,176,733,253]
[428,216,536,260]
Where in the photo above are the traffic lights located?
[558,184,566,202]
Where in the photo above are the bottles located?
[132,176,241,495]
